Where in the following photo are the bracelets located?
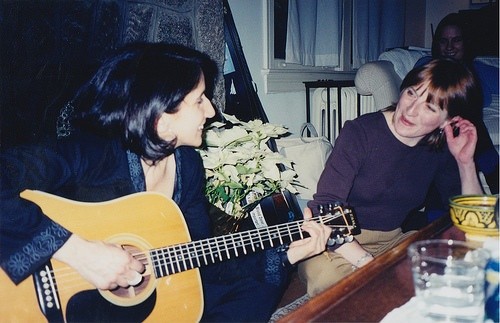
[352,251,373,273]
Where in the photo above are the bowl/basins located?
[449,194,499,236]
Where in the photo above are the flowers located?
[194,112,309,219]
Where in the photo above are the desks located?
[302,81,361,142]
[274,213,468,323]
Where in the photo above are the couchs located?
[354,48,500,154]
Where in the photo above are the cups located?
[408,239,491,323]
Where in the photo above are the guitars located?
[0,189,362,323]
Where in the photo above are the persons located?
[278,48,500,305]
[432,13,499,193]
[0,38,332,322]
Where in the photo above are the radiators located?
[312,87,376,147]
